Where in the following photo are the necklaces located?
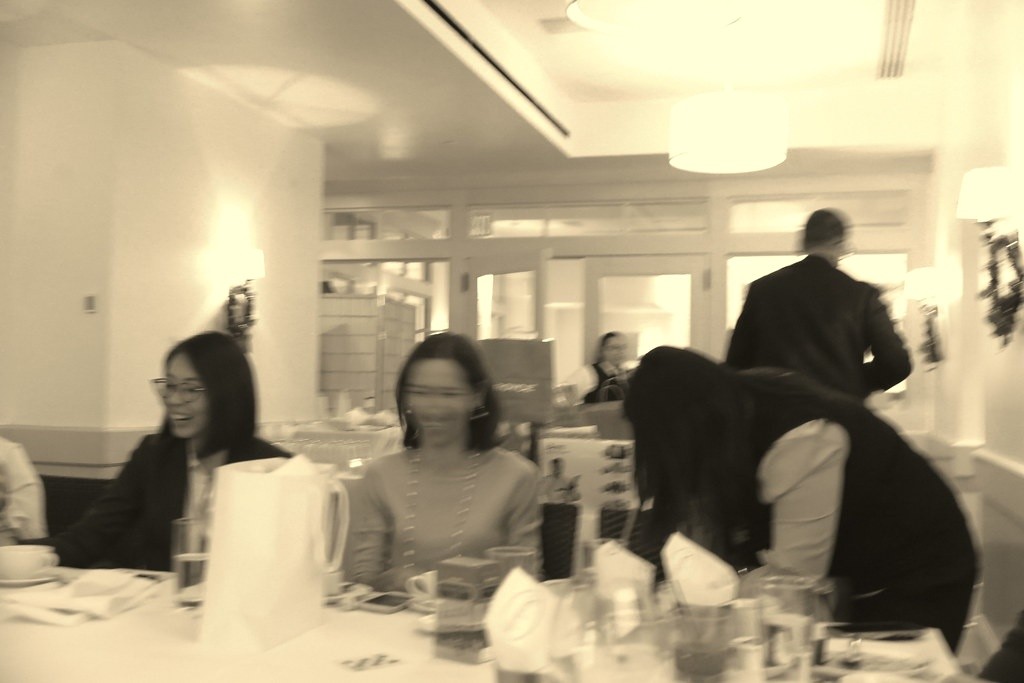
[402,447,478,589]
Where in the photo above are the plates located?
[0,577,57,586]
[327,582,373,604]
[411,603,438,613]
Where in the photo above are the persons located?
[49,331,295,570]
[619,345,983,660]
[722,207,912,404]
[0,434,49,547]
[332,332,547,604]
[570,331,633,407]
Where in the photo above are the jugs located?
[202,458,350,648]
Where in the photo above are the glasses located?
[152,378,208,404]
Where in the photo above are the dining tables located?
[0,566,957,683]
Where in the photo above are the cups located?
[406,570,437,603]
[760,575,815,613]
[172,518,210,619]
[324,572,348,592]
[483,546,540,580]
[579,540,628,567]
[674,606,732,682]
[731,636,768,673]
[729,598,766,635]
[0,545,60,578]
